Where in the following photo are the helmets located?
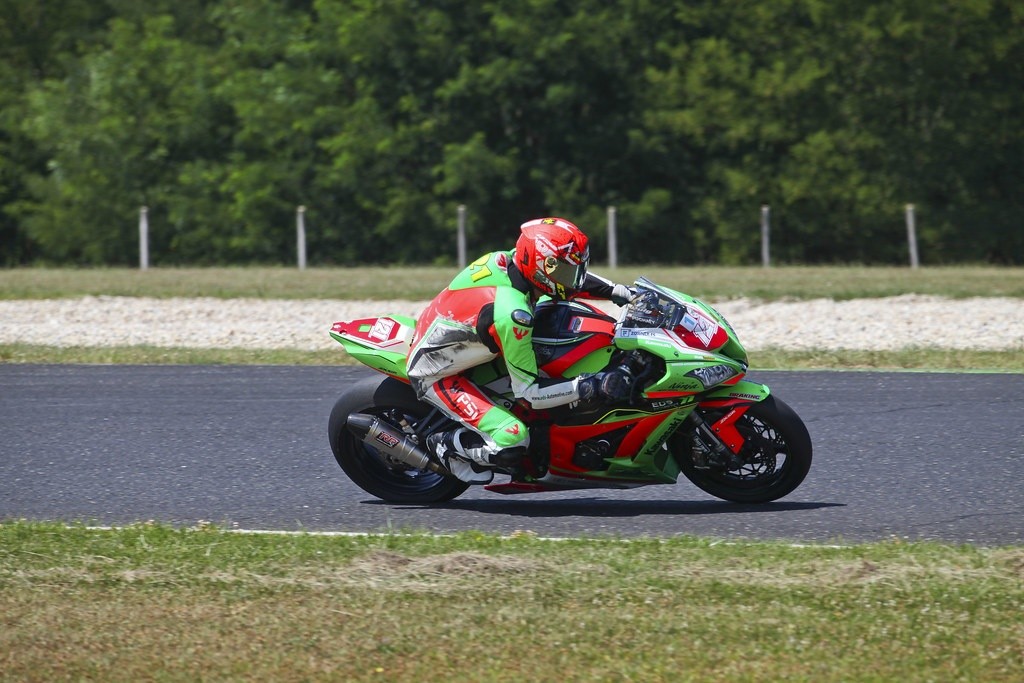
[515,217,589,302]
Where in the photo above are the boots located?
[421,428,494,485]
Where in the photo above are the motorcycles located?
[328,275,814,505]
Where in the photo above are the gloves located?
[612,285,658,307]
[578,371,630,401]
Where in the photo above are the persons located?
[406,217,659,486]
